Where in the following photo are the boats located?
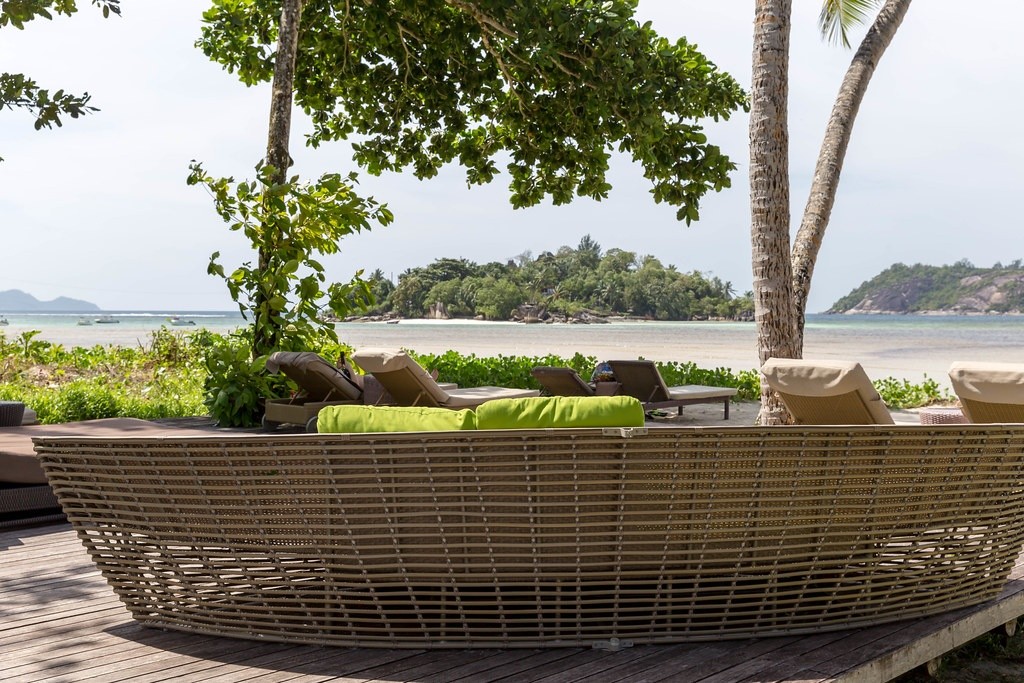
[387,320,399,324]
[0,318,9,326]
[78,317,92,326]
[94,316,120,323]
[170,317,196,326]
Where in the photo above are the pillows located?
[476,396,646,430]
[318,405,477,433]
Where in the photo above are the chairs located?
[760,357,896,426]
[0,401,269,531]
[948,359,1024,424]
[261,352,459,431]
[351,348,541,413]
[609,359,740,420]
[531,364,625,397]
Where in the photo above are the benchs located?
[31,423,1024,651]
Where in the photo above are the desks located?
[919,406,970,424]
